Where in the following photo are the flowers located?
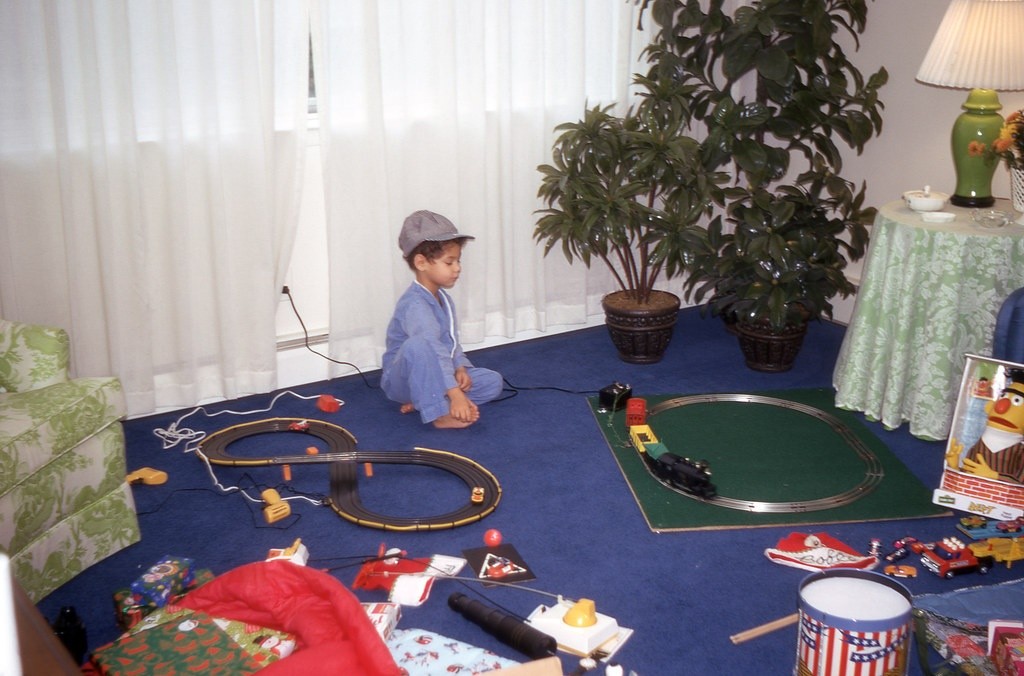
[967,110,1024,171]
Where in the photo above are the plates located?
[923,212,956,224]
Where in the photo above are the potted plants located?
[625,0,893,370]
[532,95,736,364]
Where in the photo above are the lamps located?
[916,0,1024,207]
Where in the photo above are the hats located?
[399,210,475,258]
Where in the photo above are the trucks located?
[924,538,995,580]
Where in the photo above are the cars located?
[882,535,925,583]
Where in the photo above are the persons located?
[946,369,1024,487]
[380,210,503,428]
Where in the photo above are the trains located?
[627,396,718,500]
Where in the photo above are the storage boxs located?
[361,602,402,643]
[988,620,1024,676]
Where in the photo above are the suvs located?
[996,519,1021,534]
[961,515,988,531]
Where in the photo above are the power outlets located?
[280,266,294,302]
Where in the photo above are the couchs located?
[0,320,141,606]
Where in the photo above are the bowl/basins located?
[903,186,950,214]
[972,209,1013,228]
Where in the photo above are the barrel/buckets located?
[793,568,914,676]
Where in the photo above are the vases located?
[1008,166,1024,214]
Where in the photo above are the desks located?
[832,191,1024,442]
